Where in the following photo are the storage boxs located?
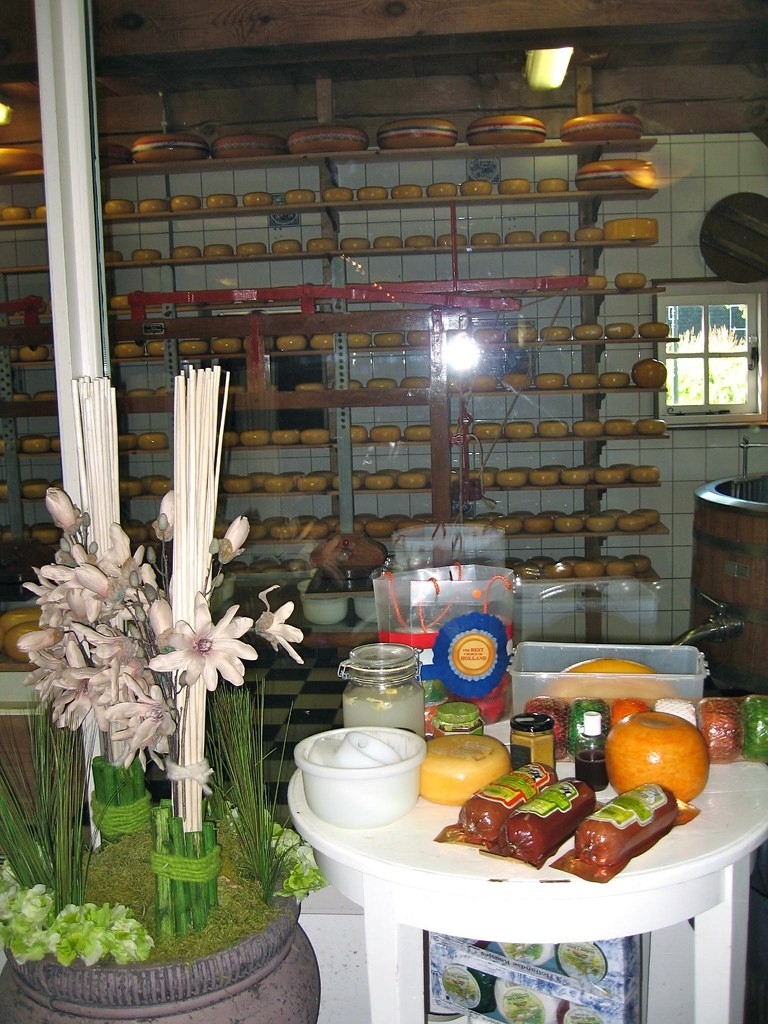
[507,641,712,762]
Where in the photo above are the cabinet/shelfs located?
[1,136,684,648]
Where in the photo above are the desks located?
[283,713,768,1024]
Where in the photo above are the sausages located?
[550,781,679,883]
[478,779,603,870]
[431,761,560,849]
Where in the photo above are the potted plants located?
[1,669,323,1023]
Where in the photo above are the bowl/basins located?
[301,598,348,625]
[353,597,378,623]
[293,726,427,829]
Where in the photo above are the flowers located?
[1,795,331,967]
[12,482,307,774]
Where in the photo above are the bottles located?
[575,711,609,792]
[338,643,426,739]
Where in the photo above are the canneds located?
[338,641,427,741]
[429,702,485,741]
[509,712,556,774]
